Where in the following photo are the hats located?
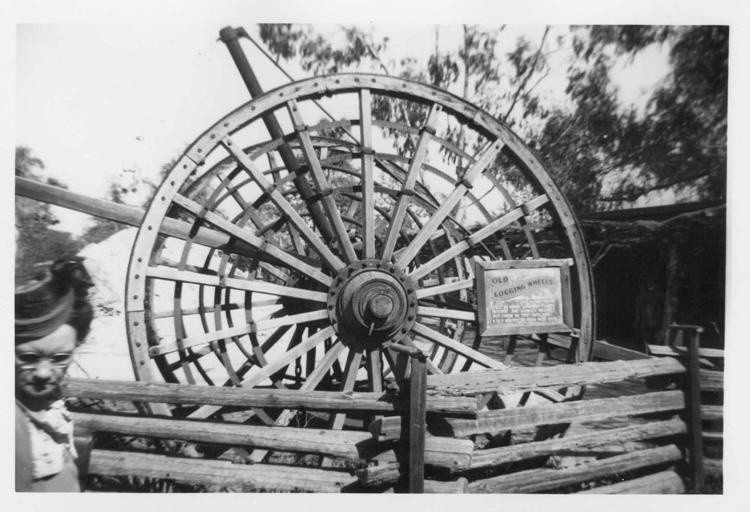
[15,268,74,339]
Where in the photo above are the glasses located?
[16,352,73,370]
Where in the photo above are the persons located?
[16,255,95,492]
